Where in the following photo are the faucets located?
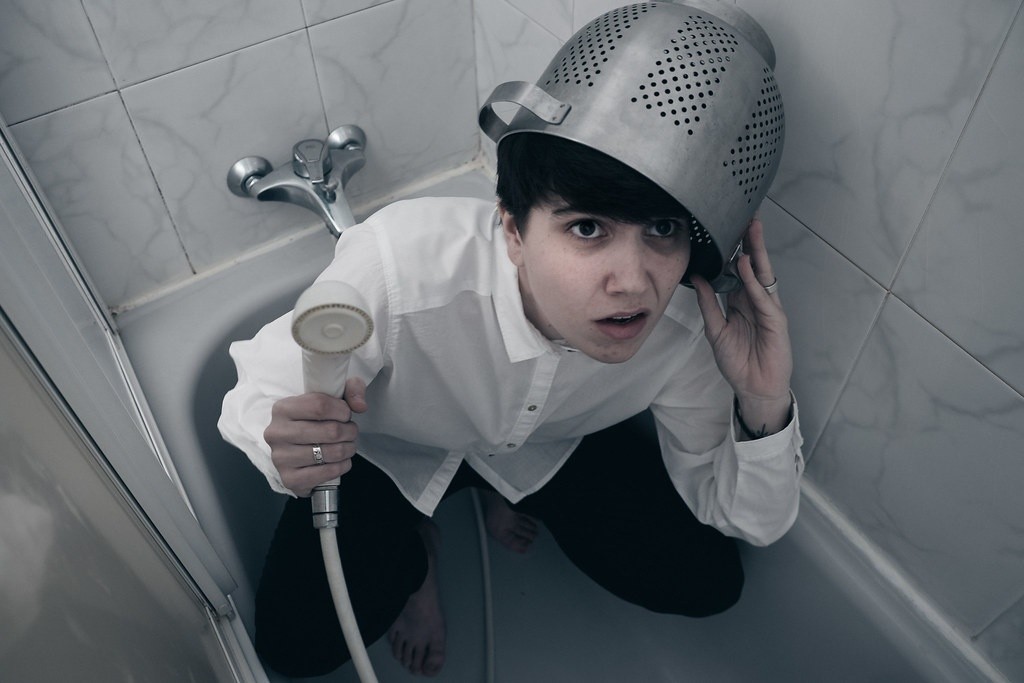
[226,123,368,240]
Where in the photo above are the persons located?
[214,133,804,683]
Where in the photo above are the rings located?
[762,275,779,292]
[312,445,325,465]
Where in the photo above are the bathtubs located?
[107,158,1009,681]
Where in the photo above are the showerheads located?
[290,279,375,515]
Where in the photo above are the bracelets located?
[734,393,759,440]
